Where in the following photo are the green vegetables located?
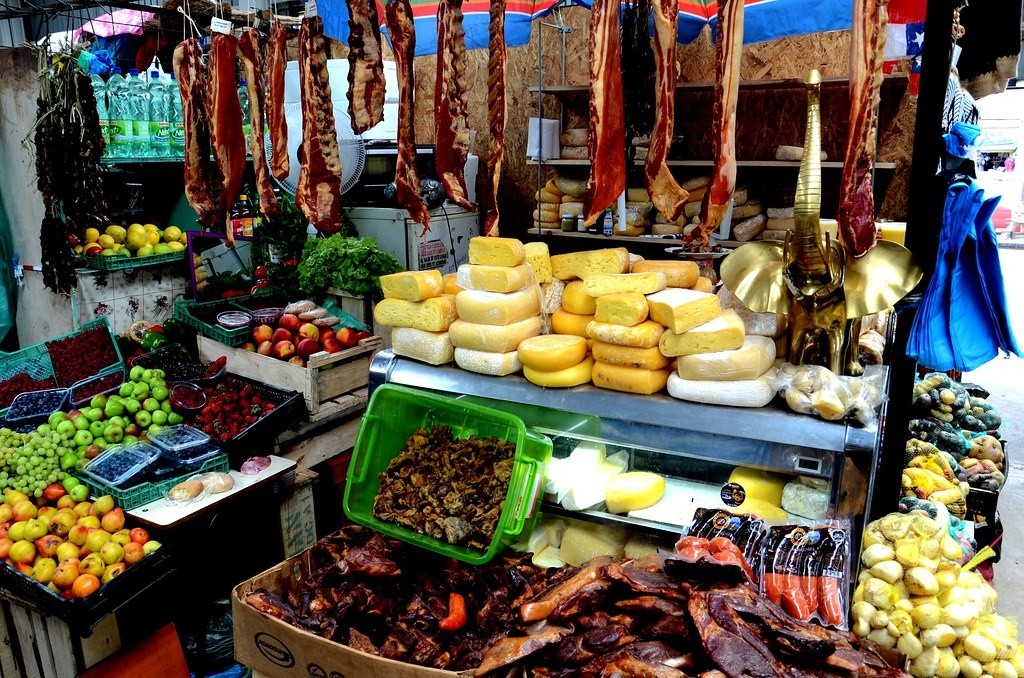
[200,193,405,302]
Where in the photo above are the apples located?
[241,315,370,369]
[49,366,184,501]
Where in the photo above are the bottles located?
[90,67,274,159]
[229,188,284,240]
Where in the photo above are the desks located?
[269,387,369,557]
[88,454,299,528]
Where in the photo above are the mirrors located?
[186,229,296,304]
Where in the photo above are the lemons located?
[75,223,188,259]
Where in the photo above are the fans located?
[264,101,367,235]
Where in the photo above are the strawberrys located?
[169,356,278,442]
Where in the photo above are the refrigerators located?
[342,204,482,276]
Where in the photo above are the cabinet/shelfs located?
[525,70,910,247]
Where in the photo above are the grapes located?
[0,427,76,504]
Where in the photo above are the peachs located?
[0,484,161,602]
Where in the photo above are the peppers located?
[115,316,193,367]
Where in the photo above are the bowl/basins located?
[216,308,285,329]
[169,381,208,415]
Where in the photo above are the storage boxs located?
[170,290,301,347]
[65,453,230,509]
[232,10,256,21]
[211,371,310,462]
[0,315,125,417]
[232,547,476,678]
[0,542,179,638]
[71,249,187,270]
[967,439,1010,520]
[343,384,555,564]
[327,287,393,348]
[197,335,384,413]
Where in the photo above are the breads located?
[531,128,842,247]
[169,480,203,499]
[193,253,209,290]
[200,472,234,494]
[374,236,793,566]
